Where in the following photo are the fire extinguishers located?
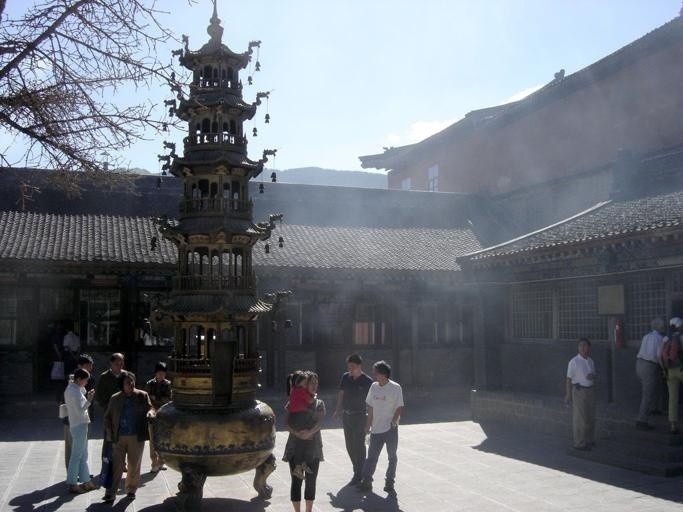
[615,318,624,351]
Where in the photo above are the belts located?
[343,408,365,416]
[640,357,657,365]
[573,382,590,390]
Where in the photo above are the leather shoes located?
[636,420,654,431]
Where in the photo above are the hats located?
[668,317,683,328]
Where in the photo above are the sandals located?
[69,480,97,493]
[291,463,316,481]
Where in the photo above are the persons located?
[636,317,666,430]
[564,338,596,450]
[286,370,318,480]
[356,359,404,490]
[284,371,326,512]
[48,323,172,501]
[657,317,683,434]
[331,354,374,485]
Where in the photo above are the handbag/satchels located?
[49,360,66,382]
[59,404,68,419]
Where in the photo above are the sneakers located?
[126,492,137,500]
[669,424,680,434]
[151,464,167,475]
[350,476,376,491]
[101,493,117,501]
[383,479,395,491]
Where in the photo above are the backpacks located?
[660,334,683,369]
